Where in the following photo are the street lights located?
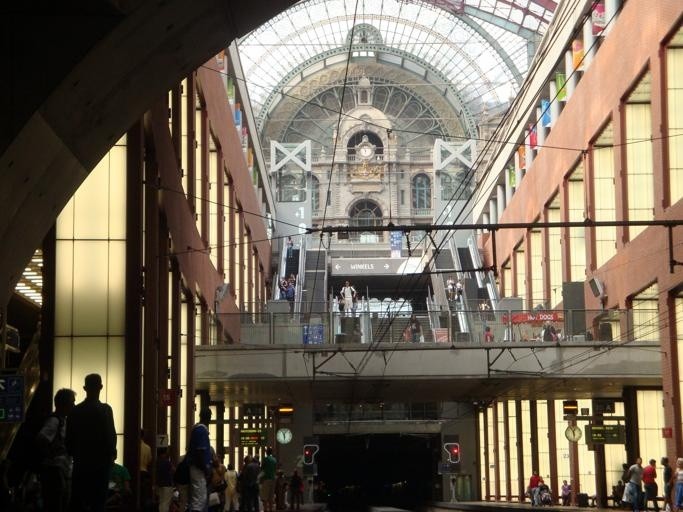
[431,196,444,238]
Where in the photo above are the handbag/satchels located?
[208,492,220,506]
[207,461,229,490]
[172,461,191,486]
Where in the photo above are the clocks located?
[276,427,293,445]
[565,424,583,443]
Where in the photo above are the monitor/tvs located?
[217,284,229,300]
[588,277,604,297]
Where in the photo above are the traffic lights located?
[442,442,460,463]
[302,444,319,465]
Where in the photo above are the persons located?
[33,390,75,511]
[611,456,683,512]
[139,422,302,512]
[277,274,295,302]
[485,326,494,344]
[287,236,294,257]
[412,314,422,344]
[540,322,558,341]
[446,276,463,302]
[65,374,117,510]
[340,281,357,316]
[527,470,571,506]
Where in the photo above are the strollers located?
[538,483,551,506]
